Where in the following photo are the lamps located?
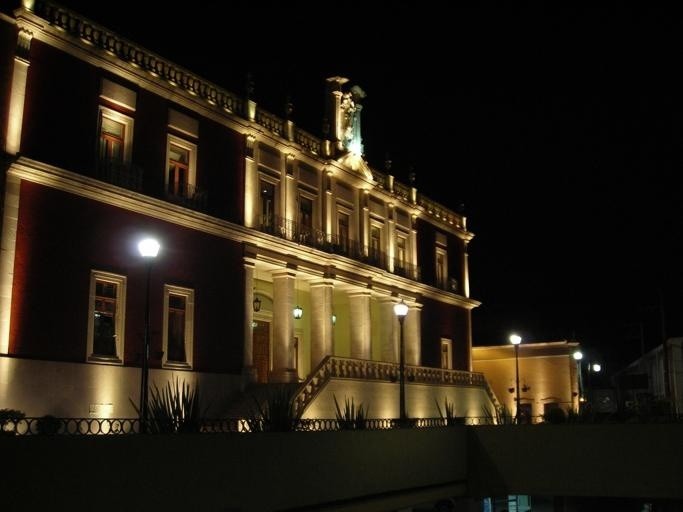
[332,288,337,328]
[253,271,261,311]
[293,278,304,319]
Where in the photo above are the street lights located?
[586,358,602,417]
[136,233,162,431]
[393,300,410,420]
[572,351,583,417]
[509,335,523,423]
